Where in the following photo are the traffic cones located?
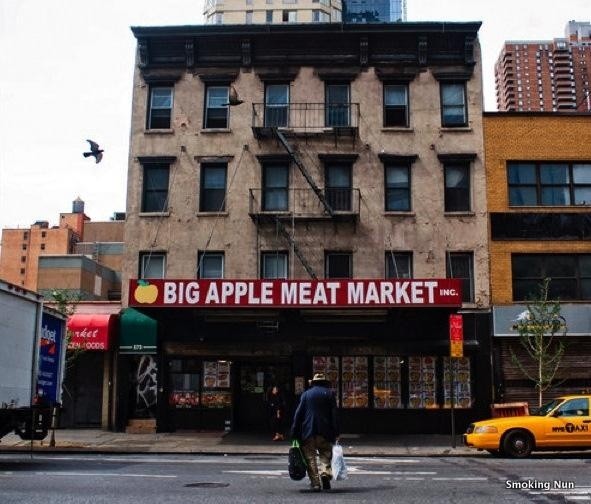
[49,429,57,447]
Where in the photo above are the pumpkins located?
[134,279,159,304]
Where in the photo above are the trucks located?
[1,277,69,441]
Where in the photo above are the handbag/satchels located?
[288,439,307,481]
[330,439,350,482]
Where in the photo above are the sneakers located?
[321,474,332,490]
[310,484,321,494]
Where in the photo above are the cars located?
[462,394,591,457]
[341,383,411,408]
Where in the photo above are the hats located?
[308,373,331,387]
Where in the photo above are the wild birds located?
[83,139,104,165]
[220,85,247,108]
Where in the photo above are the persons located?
[288,373,341,492]
